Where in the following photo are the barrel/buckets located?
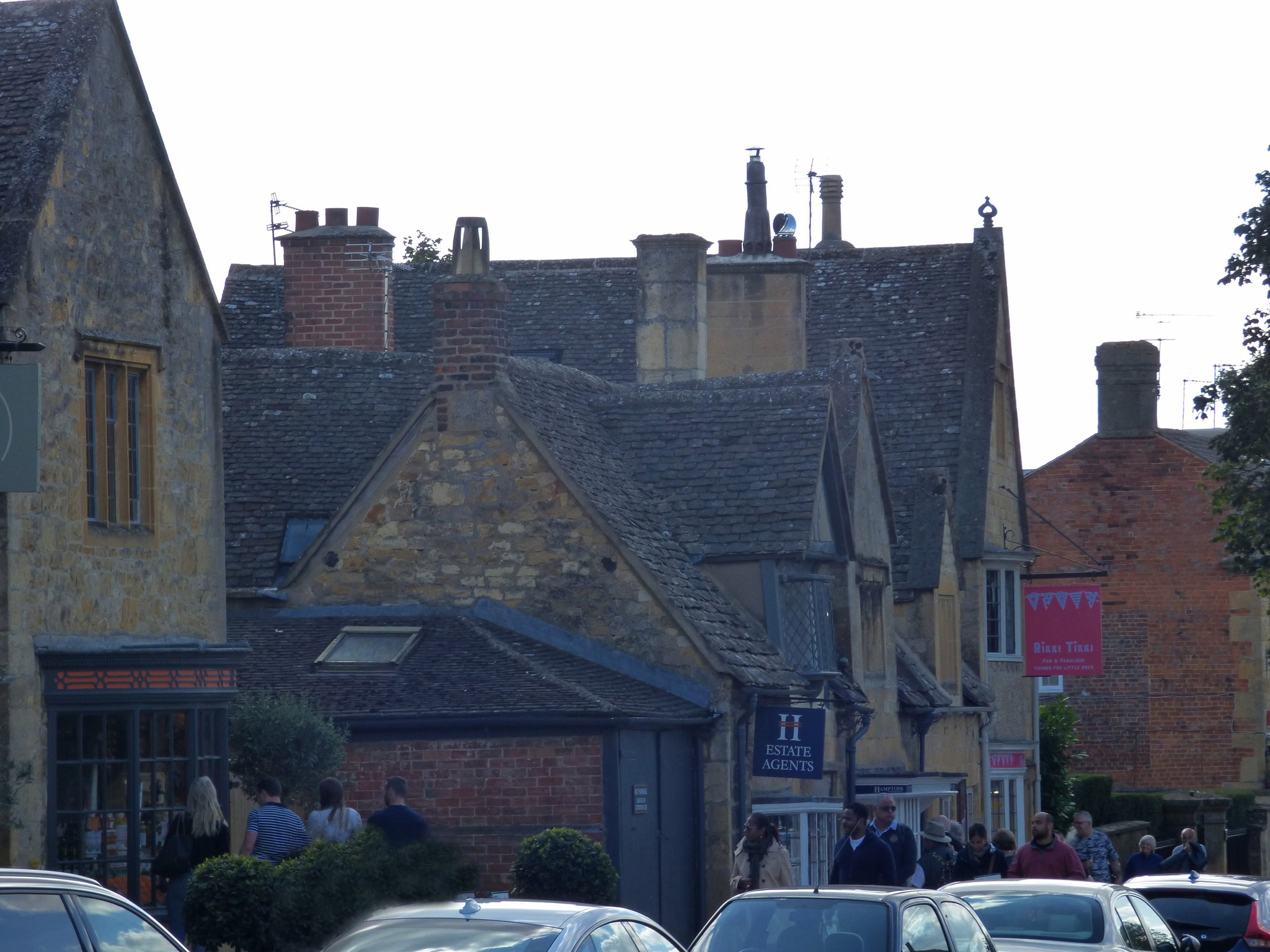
[115,825,127,840]
[84,817,102,854]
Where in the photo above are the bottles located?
[157,779,164,807]
[1088,856,1094,864]
[140,813,162,859]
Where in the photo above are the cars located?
[1122,871,1270,952]
[927,878,1202,952]
[687,885,997,952]
[320,898,685,952]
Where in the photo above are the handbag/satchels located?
[161,811,193,878]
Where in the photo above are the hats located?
[918,821,952,843]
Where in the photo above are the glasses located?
[744,824,759,830]
[877,805,897,811]
[1075,821,1089,825]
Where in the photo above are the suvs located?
[0,867,188,952]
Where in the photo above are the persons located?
[308,778,363,844]
[1124,835,1164,879]
[240,776,309,866]
[829,803,897,886]
[150,775,230,952]
[910,815,1019,891]
[1007,812,1086,881]
[367,775,430,848]
[1053,830,1094,882]
[1068,811,1122,885]
[729,812,796,897]
[1172,828,1207,872]
[866,793,917,887]
